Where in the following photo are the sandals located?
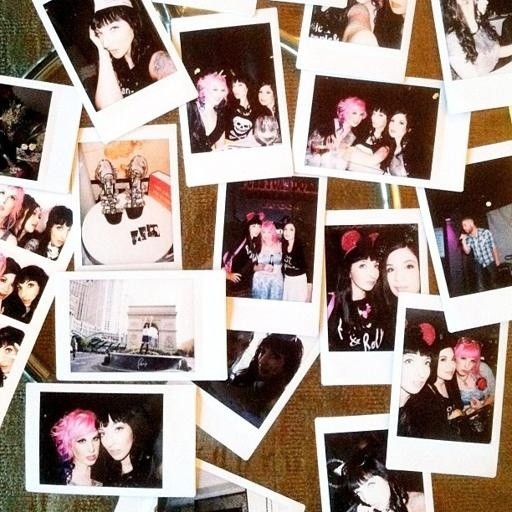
[121,155,148,219]
[95,158,123,224]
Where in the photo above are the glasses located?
[274,334,302,363]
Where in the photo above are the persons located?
[0,0,511,512]
